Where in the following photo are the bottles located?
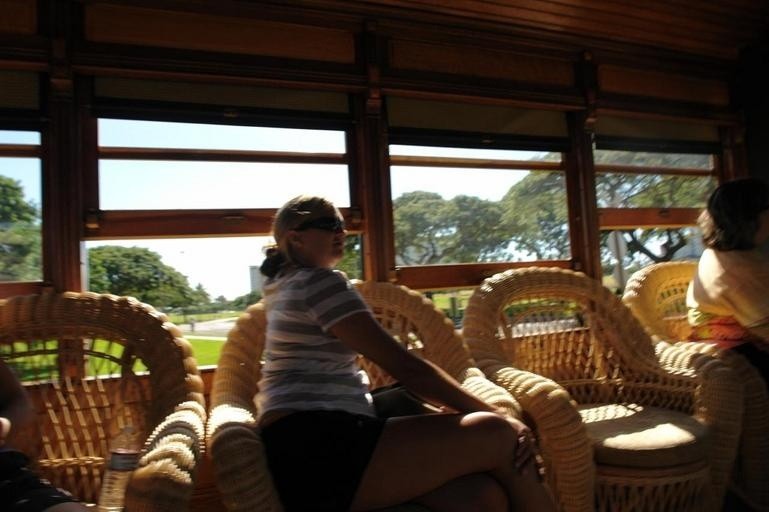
[100,424,140,510]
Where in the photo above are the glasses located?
[291,220,345,233]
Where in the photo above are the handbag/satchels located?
[370,383,443,415]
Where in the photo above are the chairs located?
[206,279,524,511]
[622,260,768,511]
[462,266,745,511]
[0,291,206,512]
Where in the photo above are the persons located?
[258,195,557,512]
[0,358,95,512]
[686,180,769,393]
